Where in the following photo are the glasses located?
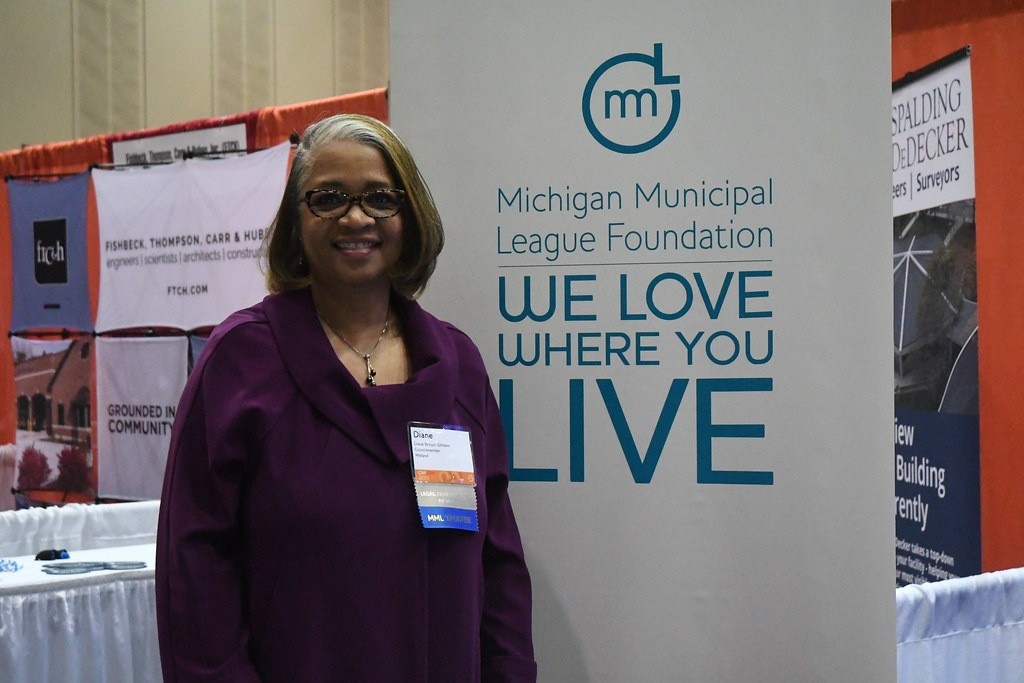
[298,188,407,220]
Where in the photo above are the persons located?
[152,112,541,681]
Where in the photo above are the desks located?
[0,542,163,683]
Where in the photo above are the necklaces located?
[314,307,393,386]
